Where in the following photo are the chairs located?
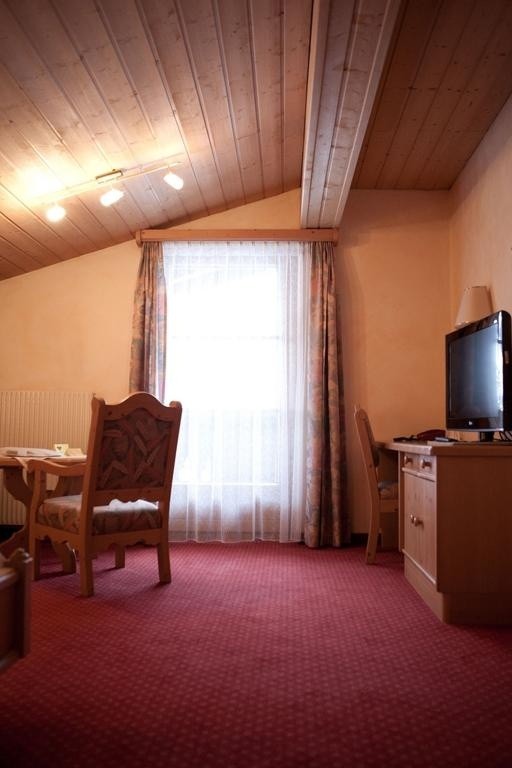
[353,405,397,566]
[27,391,184,598]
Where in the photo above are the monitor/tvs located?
[444,308,512,441]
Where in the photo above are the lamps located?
[452,283,495,332]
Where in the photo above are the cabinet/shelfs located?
[396,452,512,627]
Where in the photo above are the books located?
[49,455,88,465]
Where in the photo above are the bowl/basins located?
[54,444,68,454]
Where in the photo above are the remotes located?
[434,436,457,441]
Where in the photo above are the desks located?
[1,448,88,575]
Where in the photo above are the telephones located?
[417,429,445,441]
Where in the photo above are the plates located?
[0,446,62,458]
